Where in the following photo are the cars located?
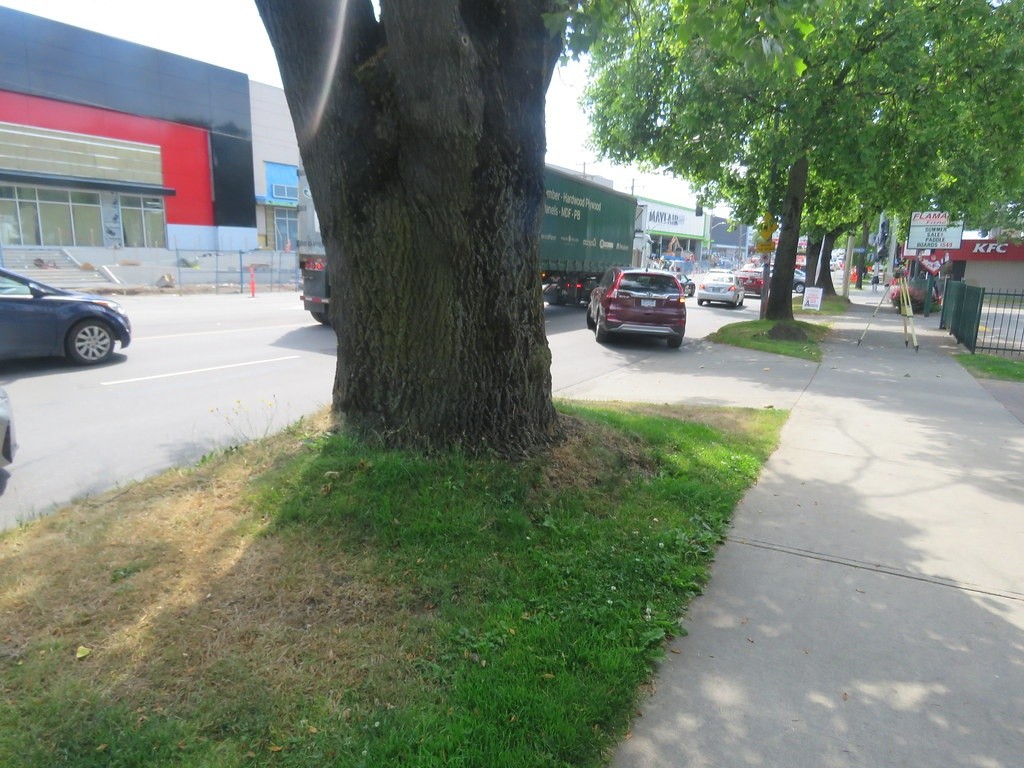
[0,266,134,367]
[0,382,17,498]
[705,269,733,280]
[734,256,809,298]
[669,271,696,296]
[696,273,746,308]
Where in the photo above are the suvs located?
[586,265,687,348]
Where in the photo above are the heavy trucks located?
[293,133,656,328]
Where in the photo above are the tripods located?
[857,271,919,352]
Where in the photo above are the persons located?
[651,258,681,273]
[871,257,881,292]
